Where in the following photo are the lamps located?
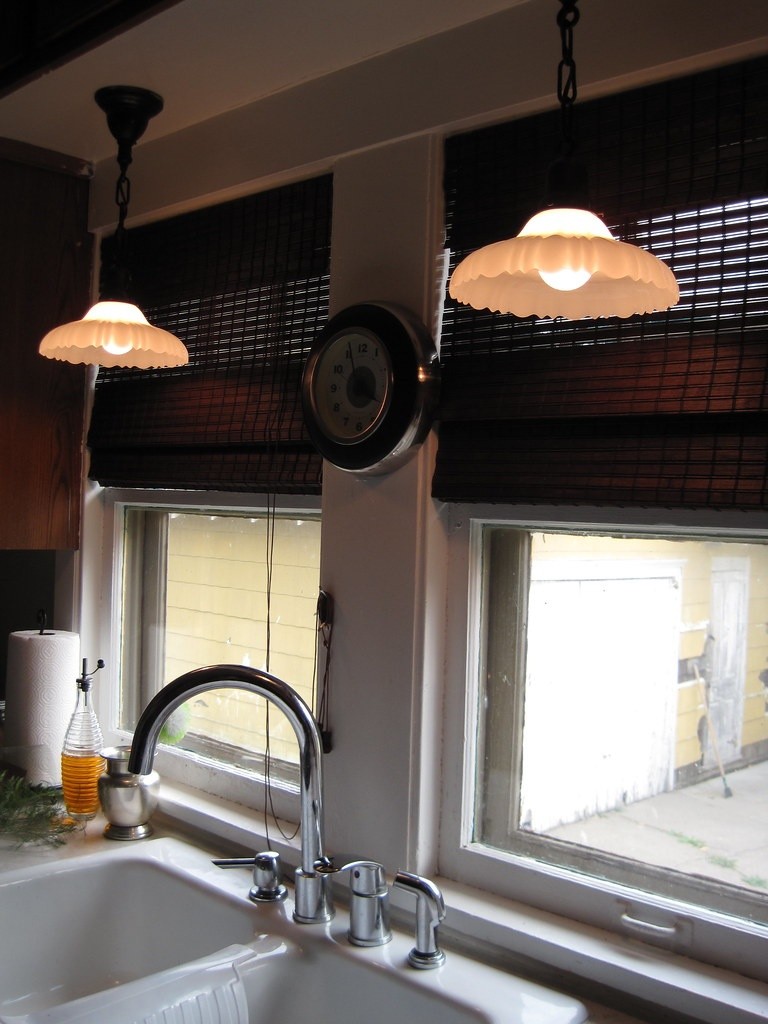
[448,0,681,320]
[37,84,188,370]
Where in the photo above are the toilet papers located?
[6,628,81,786]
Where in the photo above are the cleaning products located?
[60,658,106,817]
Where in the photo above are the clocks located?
[302,302,437,478]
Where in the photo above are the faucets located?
[125,660,336,924]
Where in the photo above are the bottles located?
[60,657,105,821]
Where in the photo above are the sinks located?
[76,937,589,1024]
[0,854,278,1024]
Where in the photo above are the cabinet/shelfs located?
[0,138,91,553]
